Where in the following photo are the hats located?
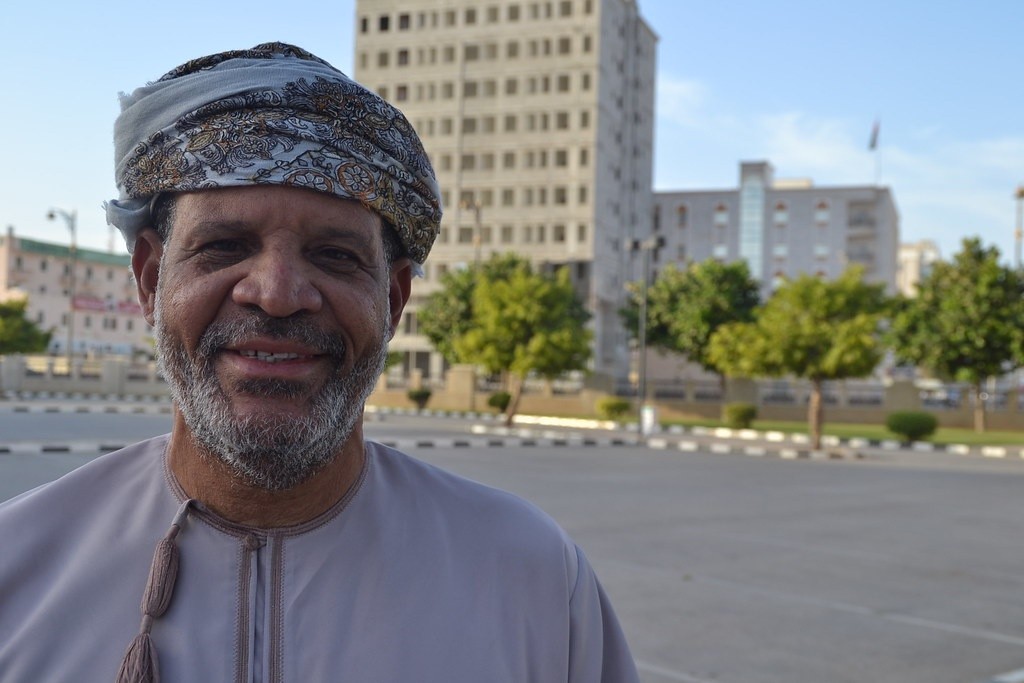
[106,40,443,266]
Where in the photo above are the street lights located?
[46,209,76,387]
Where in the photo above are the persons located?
[0,41,638,683]
[640,400,656,437]
